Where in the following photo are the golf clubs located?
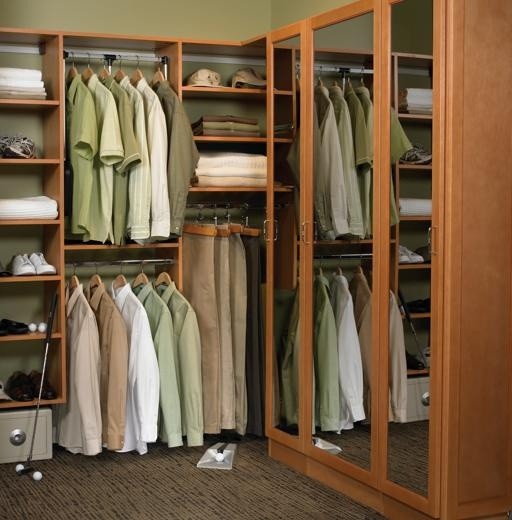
[18,294,57,473]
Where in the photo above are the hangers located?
[65,49,168,86]
[68,260,173,291]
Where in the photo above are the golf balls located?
[38,323,47,332]
[33,472,42,480]
[16,464,23,473]
[29,323,36,332]
[215,453,224,461]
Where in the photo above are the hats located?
[232,68,267,89]
[185,67,225,88]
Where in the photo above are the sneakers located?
[406,347,430,370]
[0,136,37,159]
[29,253,57,275]
[0,387,12,402]
[11,253,36,276]
[398,243,432,264]
[399,144,431,165]
[0,262,10,277]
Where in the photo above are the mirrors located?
[270,2,440,507]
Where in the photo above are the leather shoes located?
[1,318,28,336]
[5,372,34,401]
[400,298,430,313]
[29,370,57,400]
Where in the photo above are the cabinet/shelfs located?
[180,42,272,191]
[0,26,70,408]
[60,35,186,249]
[262,1,512,520]
